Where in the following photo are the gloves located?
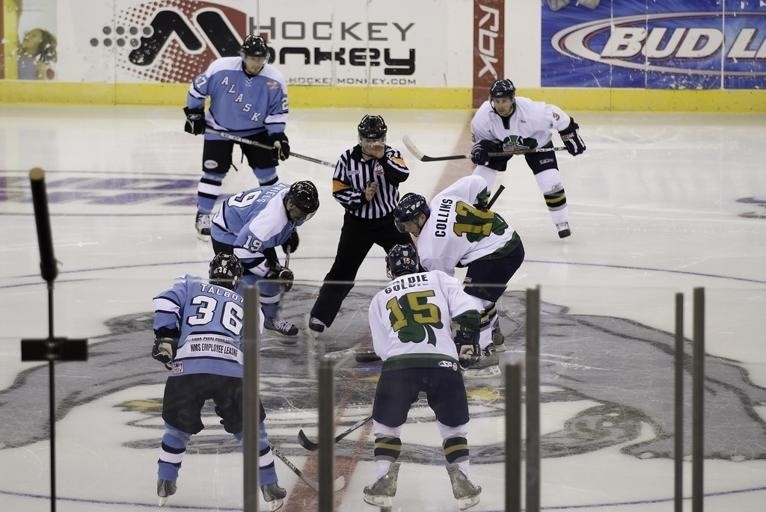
[559,118,587,155]
[455,331,481,369]
[470,144,486,165]
[269,133,291,161]
[242,34,268,56]
[183,106,207,135]
[152,331,175,369]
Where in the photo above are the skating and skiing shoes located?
[362,463,400,512]
[309,316,324,338]
[263,319,299,347]
[445,463,481,510]
[556,221,572,241]
[156,480,177,506]
[195,212,211,241]
[260,484,286,512]
[492,325,507,353]
[466,346,501,378]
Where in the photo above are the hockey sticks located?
[204,127,337,169]
[402,135,569,162]
[273,244,300,346]
[267,440,346,493]
[296,414,373,452]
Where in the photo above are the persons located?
[184,36,290,241]
[468,79,585,237]
[15,26,58,80]
[393,175,524,370]
[309,116,417,332]
[363,246,482,510]
[153,254,287,512]
[208,181,321,336]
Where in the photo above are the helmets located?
[393,192,426,233]
[290,180,320,213]
[208,251,243,290]
[385,242,419,278]
[489,78,515,98]
[357,113,387,140]
